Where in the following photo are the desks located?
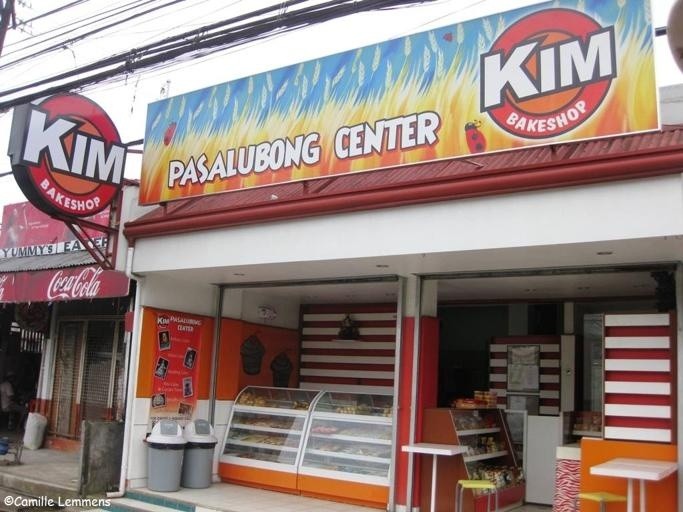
[401,442,467,511]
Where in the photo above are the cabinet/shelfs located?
[418,407,525,512]
[217,385,393,509]
[487,310,678,512]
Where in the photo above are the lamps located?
[337,304,360,340]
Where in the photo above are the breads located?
[336,403,393,417]
[232,433,300,449]
[235,415,303,433]
[271,352,293,386]
[238,390,272,408]
[311,424,339,433]
[240,335,265,375]
[291,399,310,411]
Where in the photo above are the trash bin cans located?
[145,418,188,493]
[182,418,219,490]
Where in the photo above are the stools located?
[454,479,498,512]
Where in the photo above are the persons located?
[0,371,25,434]
